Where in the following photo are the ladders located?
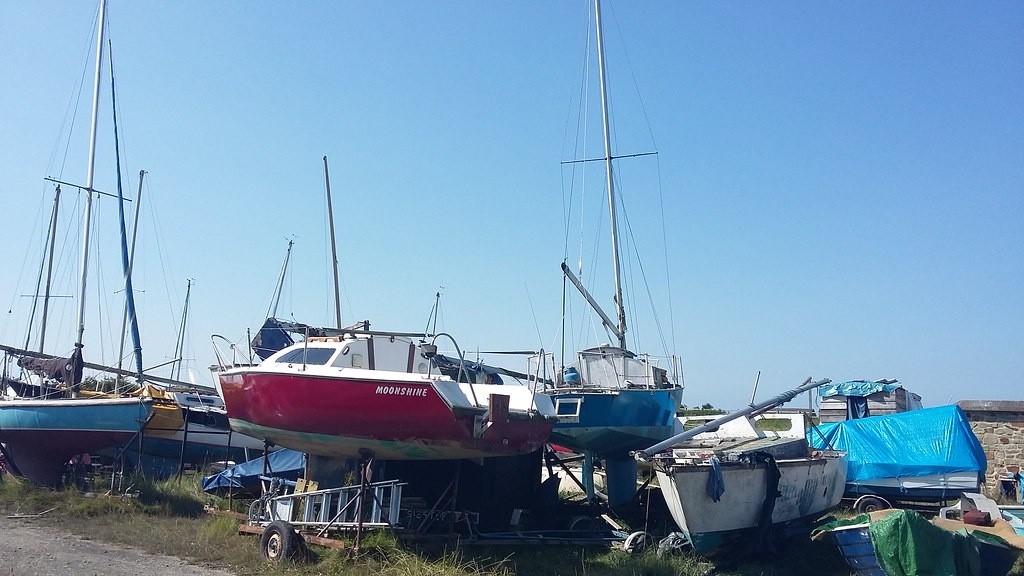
[248,478,411,535]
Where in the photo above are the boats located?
[0,0,987,562]
